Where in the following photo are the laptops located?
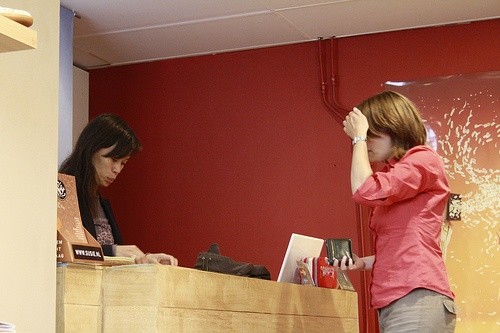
[276,233,324,284]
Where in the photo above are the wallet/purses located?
[326,238,353,265]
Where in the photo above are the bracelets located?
[145,252,151,257]
[351,137,368,145]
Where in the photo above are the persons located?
[324,90,458,333]
[59,113,179,265]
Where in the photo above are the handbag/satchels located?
[194,244,270,279]
